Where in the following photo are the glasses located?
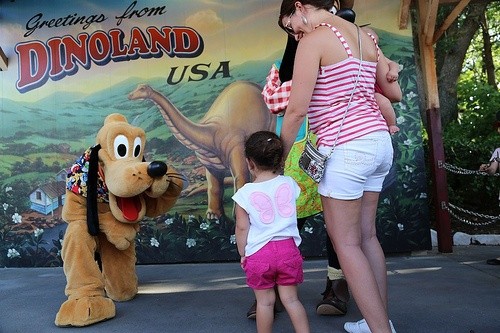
[285,8,295,35]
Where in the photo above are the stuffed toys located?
[54,113,184,328]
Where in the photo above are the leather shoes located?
[248,285,282,321]
[317,267,349,317]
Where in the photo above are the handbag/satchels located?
[298,140,328,184]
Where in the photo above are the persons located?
[278,0,404,333]
[231,131,311,333]
[359,26,401,135]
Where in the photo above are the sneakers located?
[344,318,396,333]
